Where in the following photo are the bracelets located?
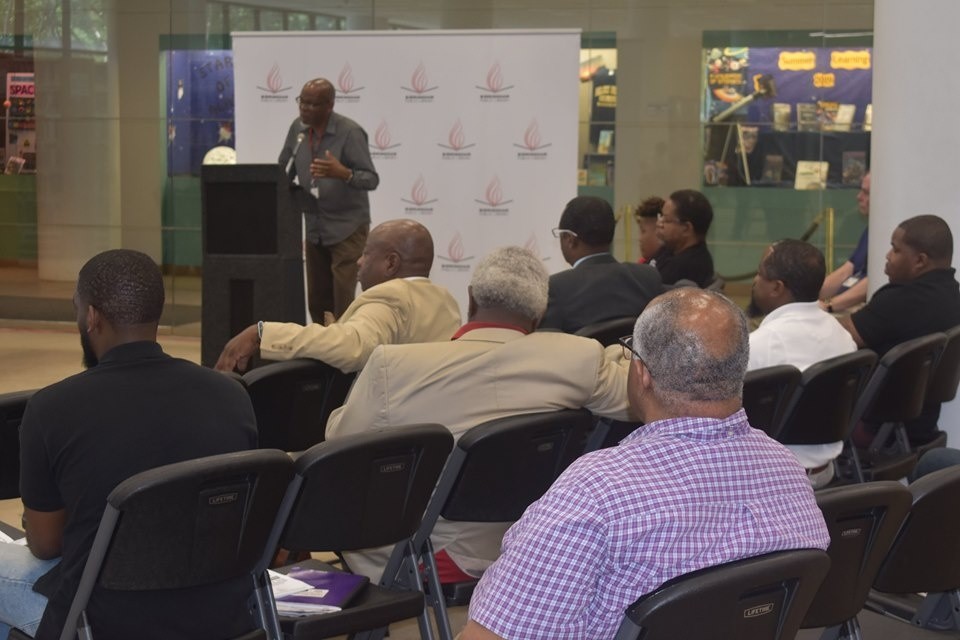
[823,297,834,313]
[345,169,353,184]
[258,321,263,339]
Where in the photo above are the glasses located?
[657,213,696,224]
[295,96,330,108]
[619,335,653,377]
[552,227,579,238]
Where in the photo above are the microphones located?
[286,133,305,175]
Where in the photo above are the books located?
[275,564,371,612]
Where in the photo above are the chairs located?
[0,317,960,640]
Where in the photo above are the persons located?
[454,288,830,639]
[211,218,462,568]
[833,214,959,464]
[322,245,644,640]
[277,77,380,322]
[817,172,870,315]
[0,248,260,640]
[538,196,665,349]
[633,189,715,290]
[741,239,861,491]
